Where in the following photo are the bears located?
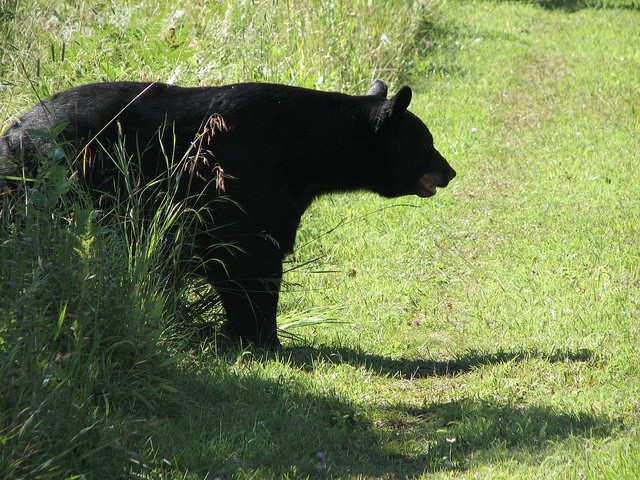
[0,80,456,352]
